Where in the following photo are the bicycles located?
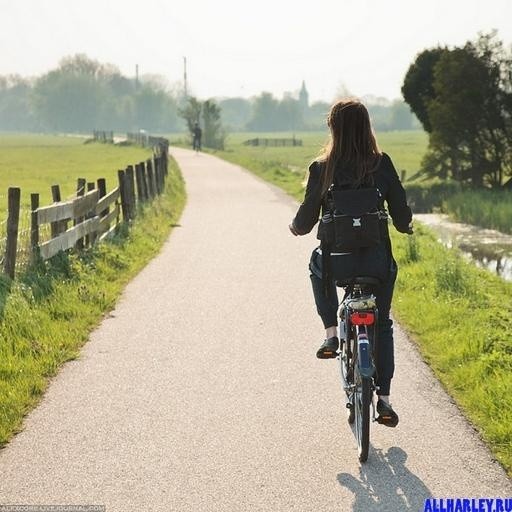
[288,211,416,463]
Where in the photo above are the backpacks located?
[333,187,381,248]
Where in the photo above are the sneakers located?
[317,337,338,359]
[376,400,398,427]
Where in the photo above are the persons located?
[288,97,412,427]
[191,121,202,151]
